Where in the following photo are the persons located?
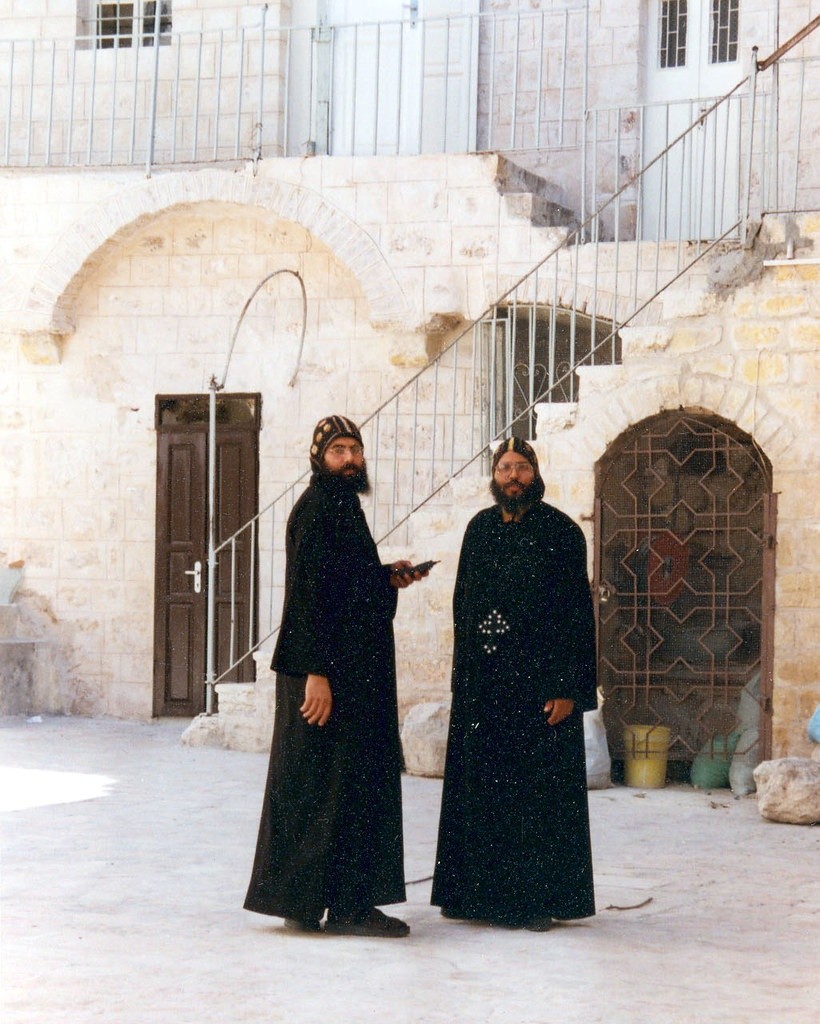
[244,415,428,941]
[432,437,598,933]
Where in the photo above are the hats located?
[309,415,363,473]
[491,436,543,479]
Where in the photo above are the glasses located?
[327,445,364,457]
[494,462,534,474]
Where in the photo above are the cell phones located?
[396,560,442,579]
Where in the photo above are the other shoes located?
[324,904,411,938]
[523,915,558,931]
[441,907,469,919]
[283,915,316,932]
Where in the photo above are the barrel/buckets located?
[623,724,672,789]
